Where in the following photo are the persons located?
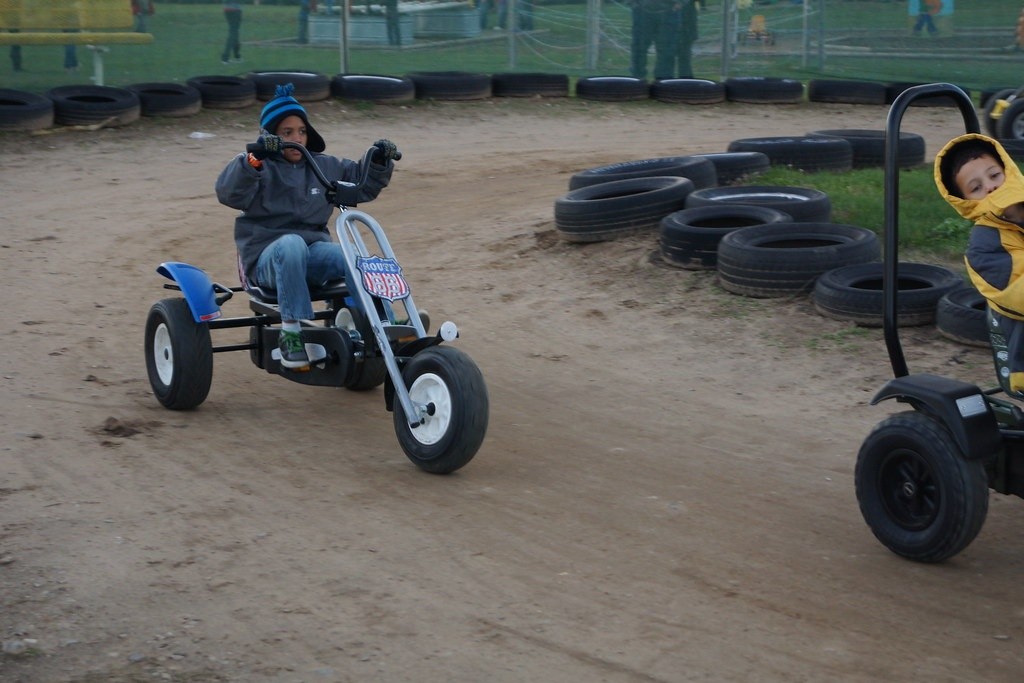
[386,0,401,45]
[216,82,398,368]
[132,0,154,32]
[220,0,247,65]
[913,0,939,36]
[630,0,700,79]
[62,27,81,68]
[477,0,534,30]
[298,0,319,43]
[934,133,1024,391]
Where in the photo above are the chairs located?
[236,248,351,305]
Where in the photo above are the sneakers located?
[394,309,430,333]
[278,331,309,369]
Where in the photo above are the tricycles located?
[144,141,489,473]
[853,81,1023,563]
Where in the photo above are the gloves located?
[253,134,285,162]
[372,138,398,160]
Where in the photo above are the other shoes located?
[219,54,244,66]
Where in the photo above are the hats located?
[259,83,325,154]
